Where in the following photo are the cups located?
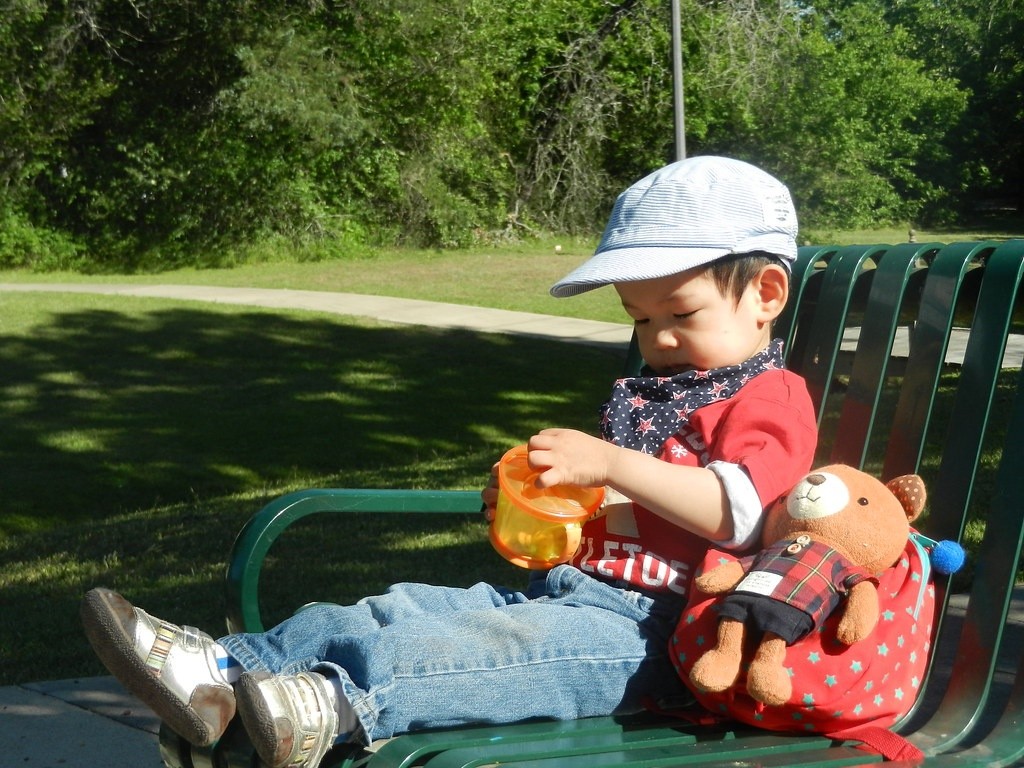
[489,442,606,570]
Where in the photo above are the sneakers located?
[82,588,236,749]
[235,671,338,768]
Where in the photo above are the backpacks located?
[663,463,965,760]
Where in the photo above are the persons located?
[80,155,818,768]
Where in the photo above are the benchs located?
[226,238,1024,768]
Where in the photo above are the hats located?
[551,156,797,298]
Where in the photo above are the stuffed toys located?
[686,463,923,703]
